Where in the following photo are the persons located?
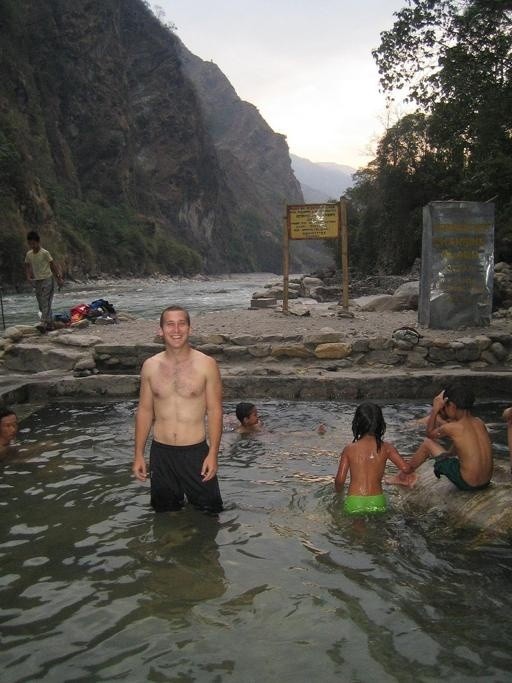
[383,379,494,491]
[233,401,326,438]
[130,303,225,514]
[500,405,512,474]
[404,400,456,430]
[0,407,21,454]
[334,402,416,520]
[23,229,64,334]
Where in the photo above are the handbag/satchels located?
[52,299,115,322]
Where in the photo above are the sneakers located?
[35,324,48,334]
